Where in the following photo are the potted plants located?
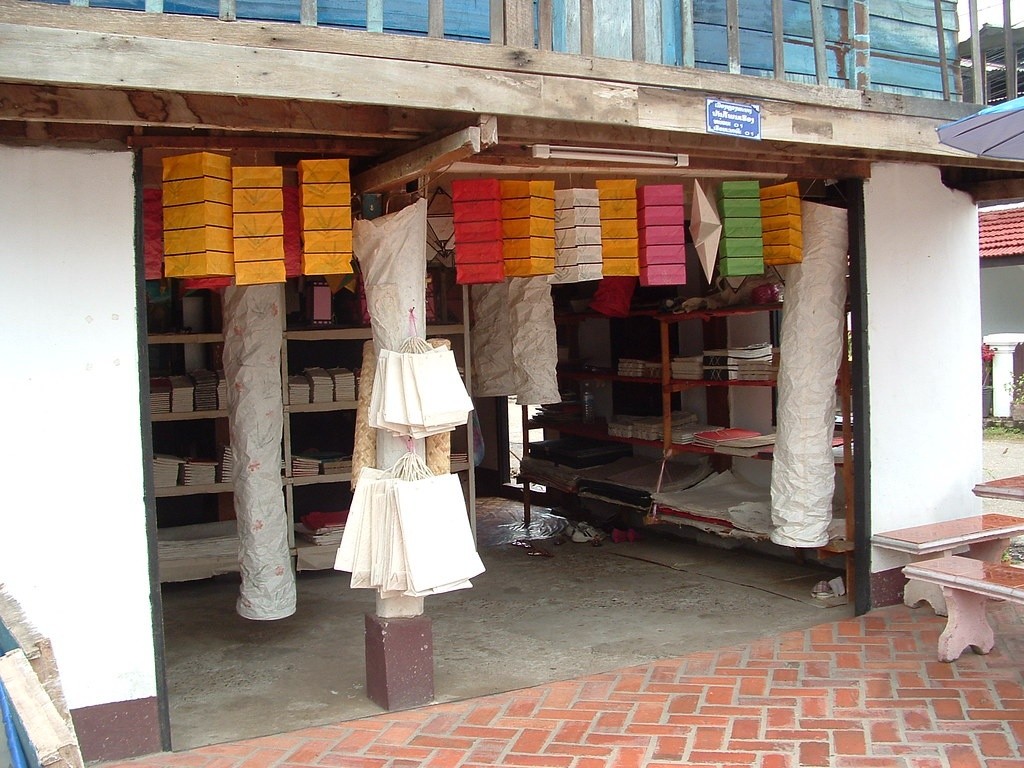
[1003,371,1024,422]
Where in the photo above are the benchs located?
[870,513,1024,617]
[901,556,1024,663]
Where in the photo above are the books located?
[288,366,360,404]
[153,442,232,486]
[617,358,662,379]
[831,435,853,464]
[291,454,352,476]
[149,368,230,413]
[608,411,775,459]
[671,342,780,381]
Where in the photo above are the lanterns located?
[421,171,806,287]
[140,151,353,293]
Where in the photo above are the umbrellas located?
[934,96,1024,162]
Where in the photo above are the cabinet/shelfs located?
[149,267,477,586]
[514,304,857,602]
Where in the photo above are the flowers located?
[982,343,998,385]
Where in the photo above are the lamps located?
[531,144,689,168]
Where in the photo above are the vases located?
[983,387,992,418]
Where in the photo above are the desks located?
[972,474,1024,503]
